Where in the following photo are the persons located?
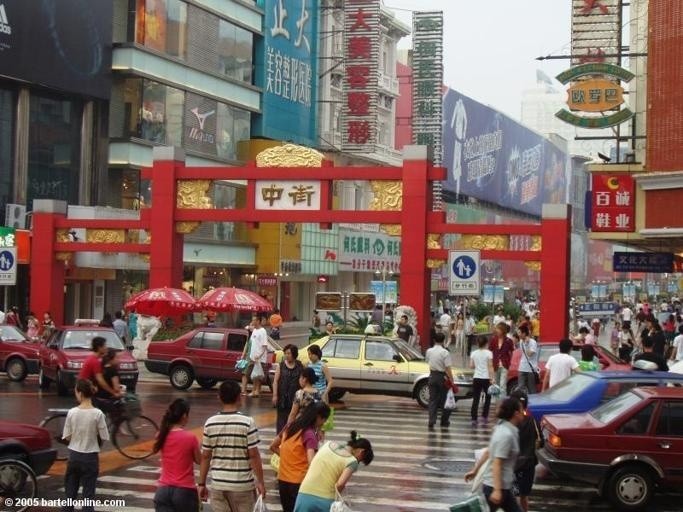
[510,390,545,512]
[139,109,154,141]
[153,399,200,512]
[197,380,266,512]
[294,431,373,512]
[150,111,166,144]
[0,296,683,447]
[463,398,524,512]
[62,378,110,511]
[268,401,330,512]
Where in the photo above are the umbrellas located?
[192,286,273,328]
[125,287,197,342]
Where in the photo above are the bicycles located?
[30,384,166,461]
[0,455,42,512]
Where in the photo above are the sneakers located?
[482,417,489,425]
[472,421,477,425]
[428,425,434,428]
[440,422,450,427]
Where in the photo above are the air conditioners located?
[5,203,26,229]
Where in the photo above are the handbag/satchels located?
[622,343,633,352]
[488,384,500,394]
[277,393,292,409]
[271,453,280,472]
[534,372,540,384]
[330,500,352,512]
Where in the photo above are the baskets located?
[121,400,140,417]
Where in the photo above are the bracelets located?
[198,483,206,487]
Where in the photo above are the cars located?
[277,333,485,411]
[142,325,305,396]
[3,319,51,386]
[535,386,675,506]
[495,366,677,430]
[0,423,59,494]
[35,318,139,395]
